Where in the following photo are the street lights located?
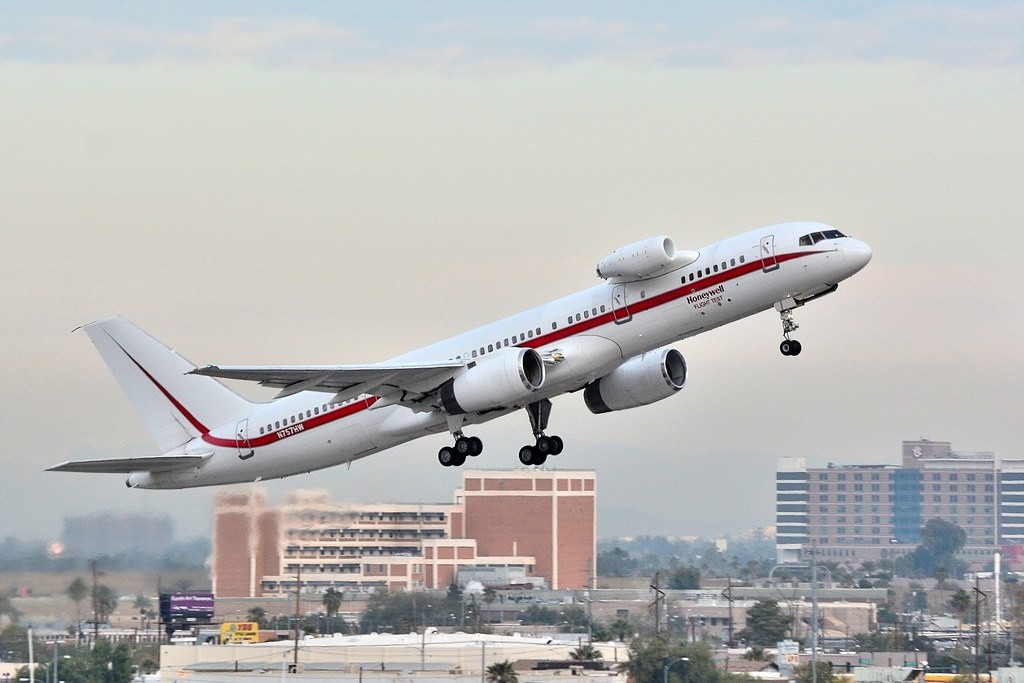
[663,656,690,683]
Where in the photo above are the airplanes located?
[44,220,874,499]
